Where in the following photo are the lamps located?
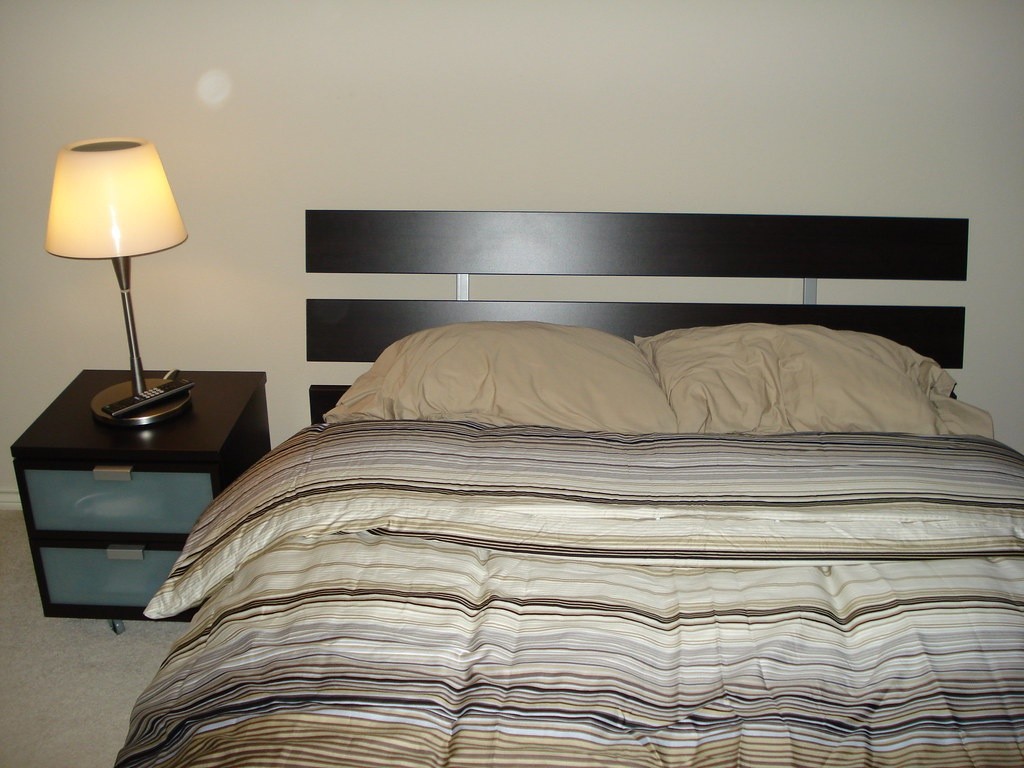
[44,136,193,426]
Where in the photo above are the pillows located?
[633,322,996,441]
[324,321,679,436]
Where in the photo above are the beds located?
[112,207,1024,768]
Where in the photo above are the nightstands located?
[10,370,272,634]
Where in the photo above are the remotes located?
[101,379,195,417]
